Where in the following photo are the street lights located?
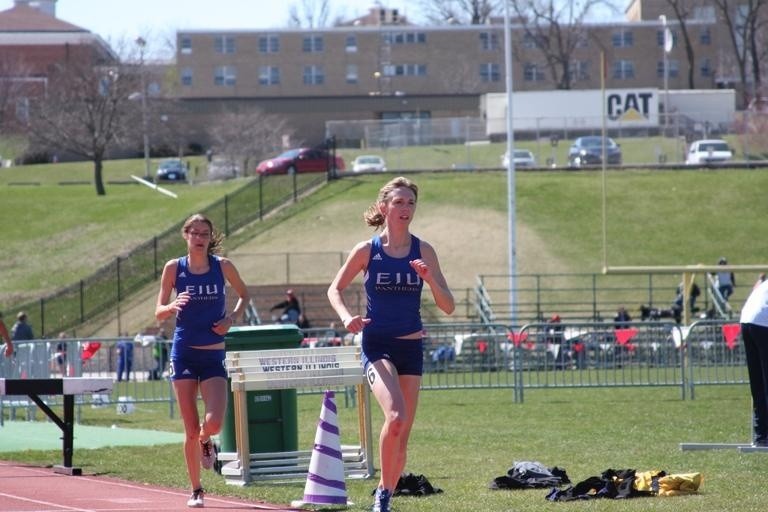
[133,36,152,183]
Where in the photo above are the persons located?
[710,257,736,301]
[739,277,767,447]
[753,273,766,292]
[326,176,455,512]
[547,313,564,344]
[614,307,631,329]
[269,288,300,324]
[295,314,309,328]
[672,281,701,312]
[0,311,168,380]
[154,213,251,509]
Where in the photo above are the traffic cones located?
[290,388,355,510]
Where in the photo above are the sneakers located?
[374,489,393,512]
[751,442,768,449]
[199,421,215,470]
[187,487,207,507]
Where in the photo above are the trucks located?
[480,85,678,147]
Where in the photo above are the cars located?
[249,145,345,177]
[206,156,240,181]
[156,154,188,181]
[499,148,536,167]
[349,153,387,172]
[680,135,734,165]
[567,134,623,165]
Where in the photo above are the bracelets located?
[227,316,233,325]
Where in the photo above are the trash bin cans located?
[220,324,304,452]
